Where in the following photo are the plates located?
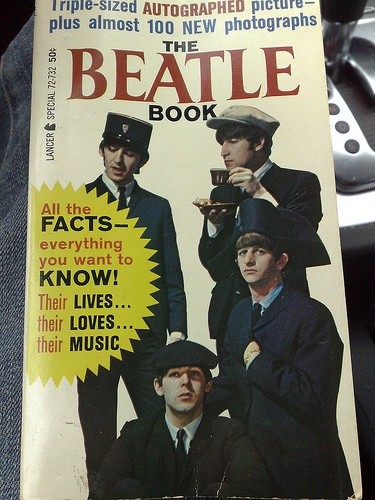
[203,203,237,211]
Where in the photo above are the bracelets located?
[245,349,261,363]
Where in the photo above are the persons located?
[203,199,355,500]
[194,105,332,419]
[88,341,278,500]
[77,113,187,471]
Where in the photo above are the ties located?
[176,429,186,466]
[252,303,262,328]
[241,189,253,201]
[118,187,127,210]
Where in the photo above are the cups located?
[210,168,230,186]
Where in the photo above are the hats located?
[147,340,218,375]
[231,198,297,259]
[206,105,280,137]
[102,112,154,151]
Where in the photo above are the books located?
[19,1,364,500]
